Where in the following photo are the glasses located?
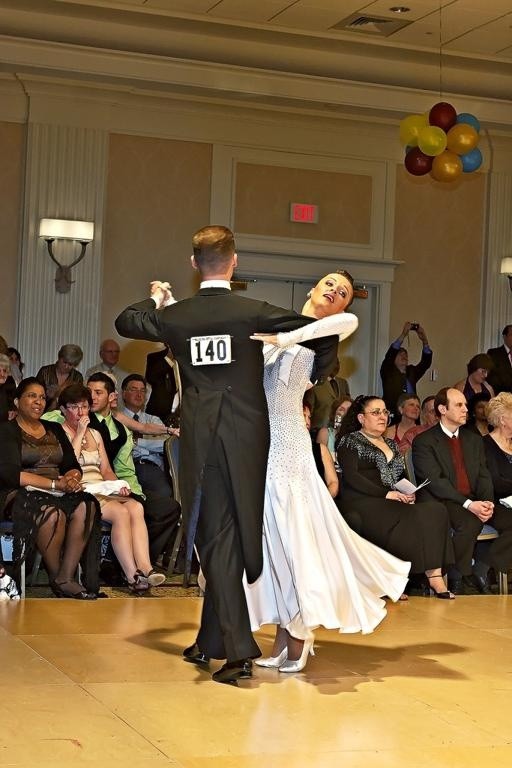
[360,410,390,417]
[66,405,90,412]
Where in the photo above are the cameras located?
[409,323,420,330]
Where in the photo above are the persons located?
[150,269,412,674]
[114,225,340,683]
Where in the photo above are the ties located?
[132,415,143,442]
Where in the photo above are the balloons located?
[400,102,483,183]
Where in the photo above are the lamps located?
[36,216,97,296]
[499,256,512,292]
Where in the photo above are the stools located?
[1,429,510,600]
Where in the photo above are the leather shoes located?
[212,660,253,682]
[254,646,289,667]
[182,643,209,664]
[448,574,493,596]
[53,578,99,600]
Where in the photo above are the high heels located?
[278,631,316,673]
[127,570,166,595]
[424,576,457,599]
